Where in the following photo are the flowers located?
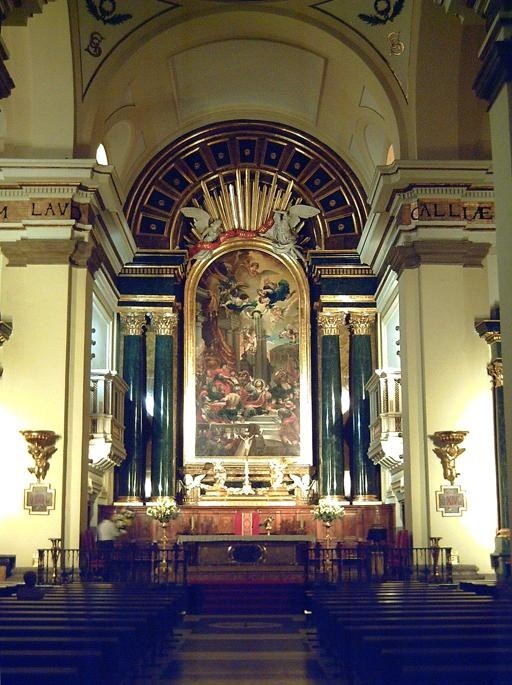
[311,494,346,522]
[147,496,180,521]
[111,507,138,535]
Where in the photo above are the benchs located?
[0,580,186,684]
[301,577,512,685]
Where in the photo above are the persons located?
[16,571,45,601]
[195,251,299,456]
[95,511,128,582]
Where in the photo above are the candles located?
[245,456,249,477]
